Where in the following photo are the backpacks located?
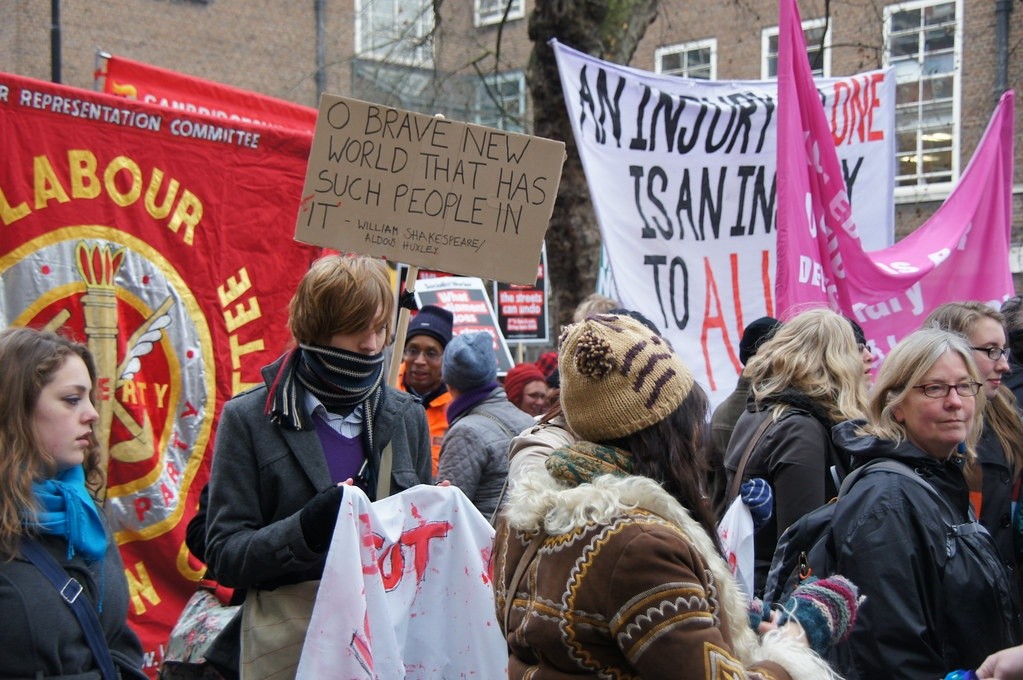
[763,458,955,680]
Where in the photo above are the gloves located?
[740,477,771,532]
[777,574,868,658]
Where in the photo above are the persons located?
[825,292,1023,680]
[0,328,150,680]
[159,484,246,680]
[206,255,450,680]
[392,301,673,525]
[491,314,866,680]
[707,316,872,598]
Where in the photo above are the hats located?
[847,317,866,344]
[404,305,454,351]
[535,352,558,376]
[557,312,695,445]
[440,331,498,388]
[739,317,782,366]
[504,362,547,407]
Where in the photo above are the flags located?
[1,57,342,680]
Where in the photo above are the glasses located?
[856,345,872,352]
[910,381,983,399]
[967,345,1010,361]
